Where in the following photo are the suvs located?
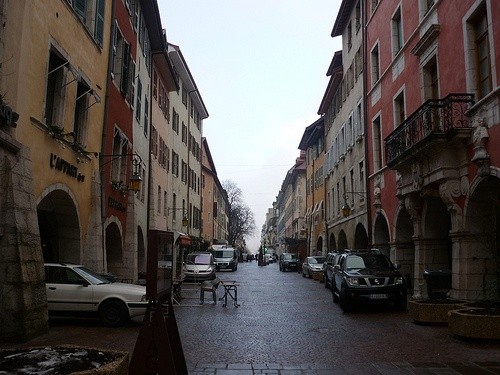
[332,249,408,314]
[323,250,341,292]
[279,253,301,272]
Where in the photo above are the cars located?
[44,261,150,327]
[302,255,327,281]
[242,249,277,265]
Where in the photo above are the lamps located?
[168,207,188,228]
[86,152,98,158]
[99,152,143,194]
[342,192,371,219]
[62,132,76,138]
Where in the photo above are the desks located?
[219,281,240,308]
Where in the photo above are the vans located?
[183,251,217,282]
[213,248,238,272]
